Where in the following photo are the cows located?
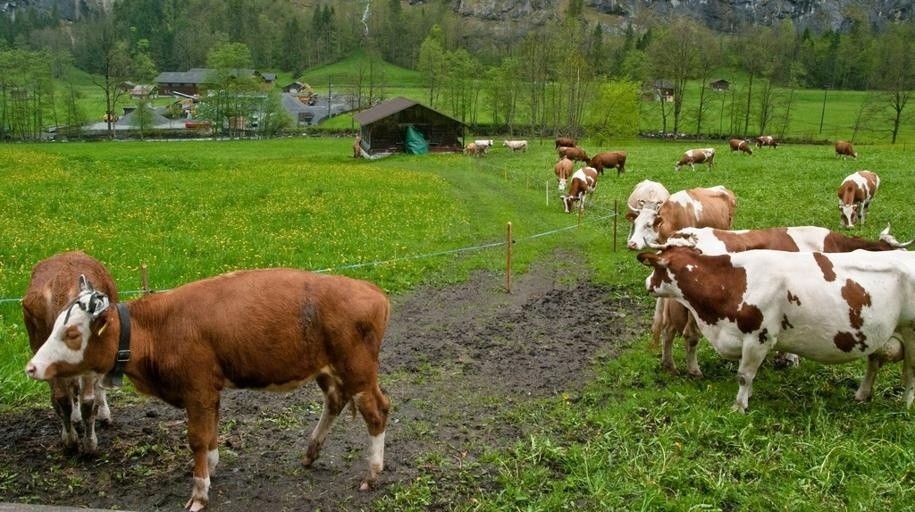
[628,180,670,213]
[554,137,626,213]
[25,268,390,512]
[837,170,880,231]
[640,234,915,415]
[502,140,528,153]
[729,139,752,155]
[628,185,737,254]
[835,140,858,161]
[651,220,915,381]
[22,252,116,455]
[675,149,714,172]
[756,136,778,150]
[463,140,493,159]
